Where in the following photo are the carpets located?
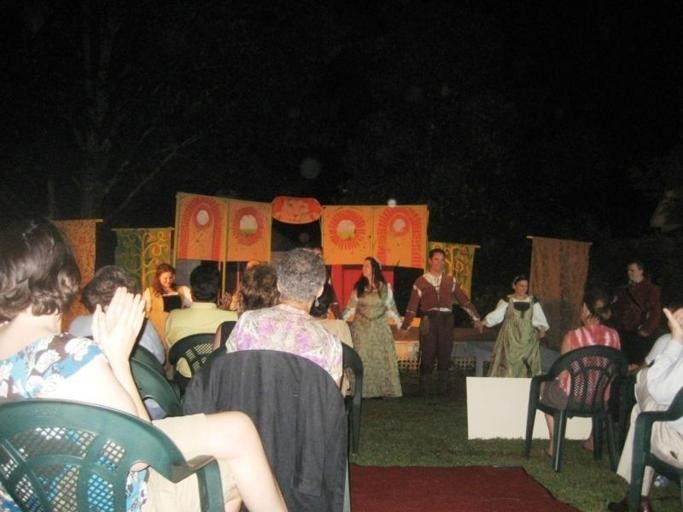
[348,464,582,512]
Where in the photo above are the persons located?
[399,248,484,398]
[476,275,550,378]
[540,261,683,511]
[345,256,401,398]
[0,211,353,511]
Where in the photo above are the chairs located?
[522,345,628,473]
[1,397,224,512]
[127,332,218,423]
[627,386,681,512]
[341,339,364,458]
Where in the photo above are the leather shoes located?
[608,494,653,512]
